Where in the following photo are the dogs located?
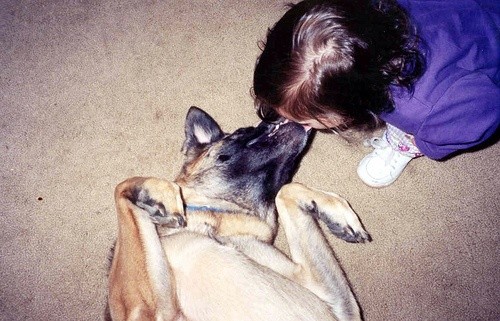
[107,104,373,321]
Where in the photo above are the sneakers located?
[356,130,414,189]
